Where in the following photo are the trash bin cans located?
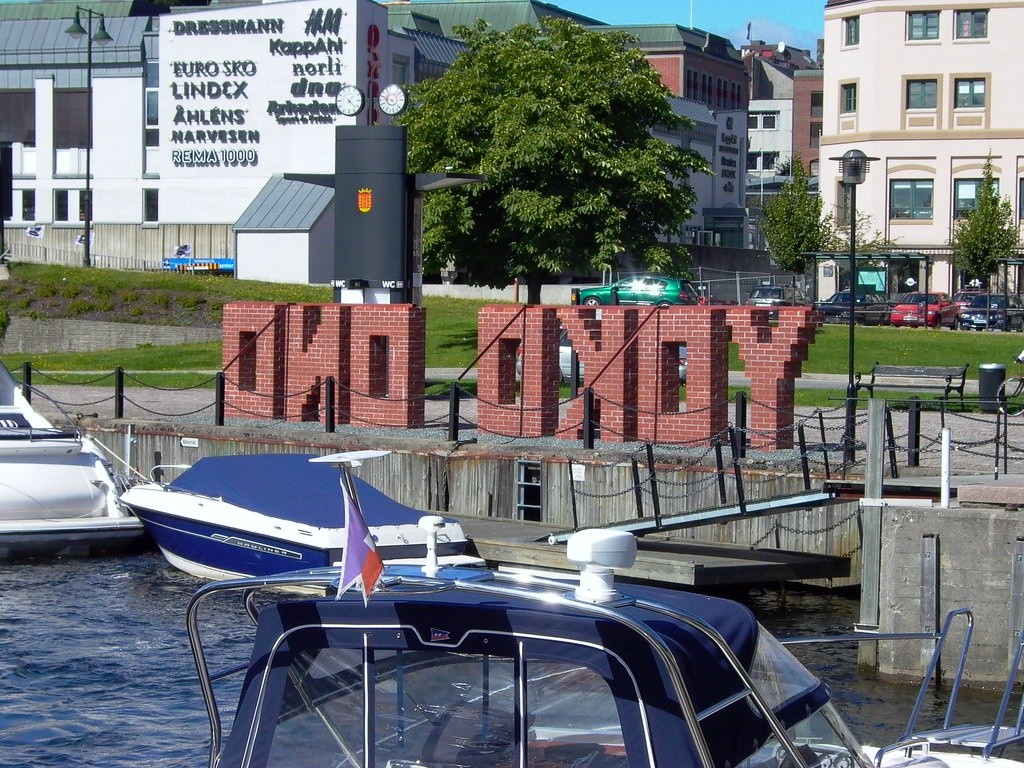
[979,362,1006,412]
[570,288,580,305]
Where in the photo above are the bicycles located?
[994,356,1024,418]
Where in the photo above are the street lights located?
[828,149,881,413]
[60,4,117,270]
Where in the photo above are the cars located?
[960,293,1024,333]
[818,287,890,326]
[745,284,815,306]
[890,289,959,331]
[517,322,688,386]
[951,288,989,323]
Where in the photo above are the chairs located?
[771,290,784,298]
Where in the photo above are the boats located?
[1,357,148,553]
[116,453,486,604]
[188,516,873,768]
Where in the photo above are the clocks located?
[336,85,365,116]
[378,83,408,116]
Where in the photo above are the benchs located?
[854,362,970,411]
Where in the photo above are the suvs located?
[579,275,701,306]
